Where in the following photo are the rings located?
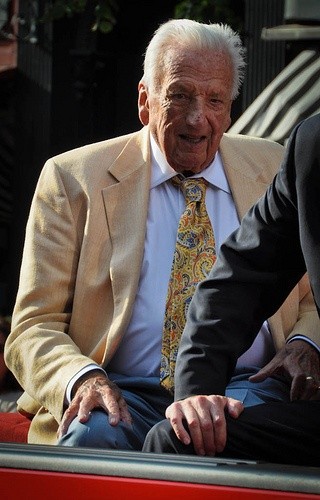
[304,376,315,384]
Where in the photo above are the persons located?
[152,113,320,468]
[4,18,320,452]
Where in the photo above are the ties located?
[158,173,217,393]
